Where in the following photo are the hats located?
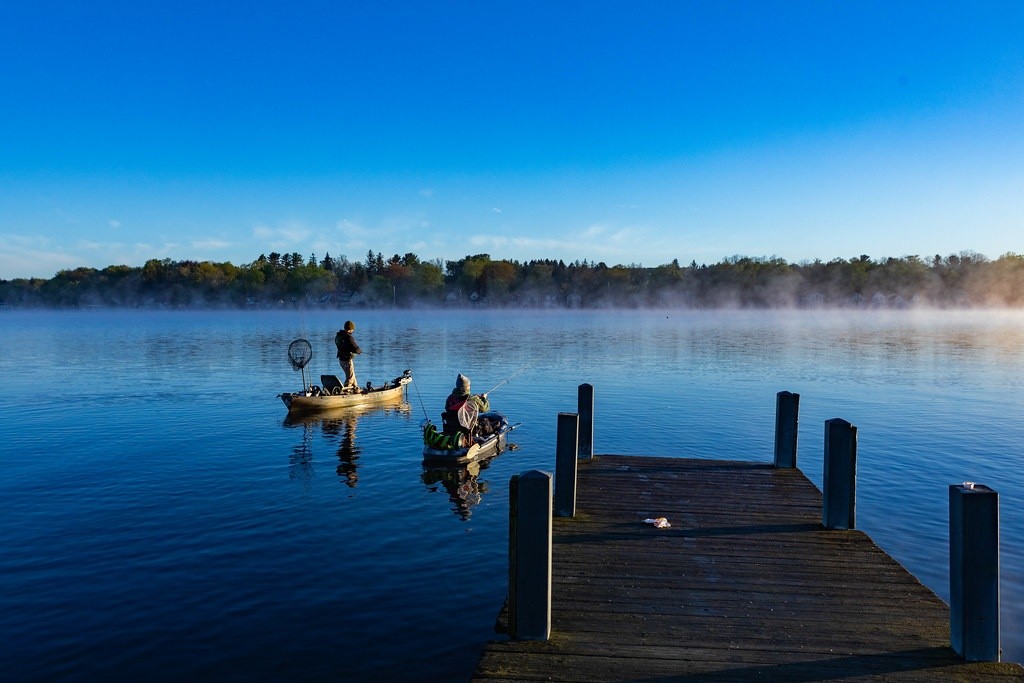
[344,321,354,330]
[456,374,471,387]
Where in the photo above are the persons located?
[441,372,493,437]
[441,468,490,522]
[336,416,364,488]
[334,321,363,395]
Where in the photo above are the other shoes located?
[354,387,360,394]
[492,421,500,429]
[343,389,351,394]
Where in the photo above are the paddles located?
[466,423,523,460]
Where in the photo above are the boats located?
[422,410,509,464]
[275,367,412,418]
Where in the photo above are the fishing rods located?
[299,304,311,388]
[480,352,550,396]
[360,351,393,363]
[406,357,431,425]
